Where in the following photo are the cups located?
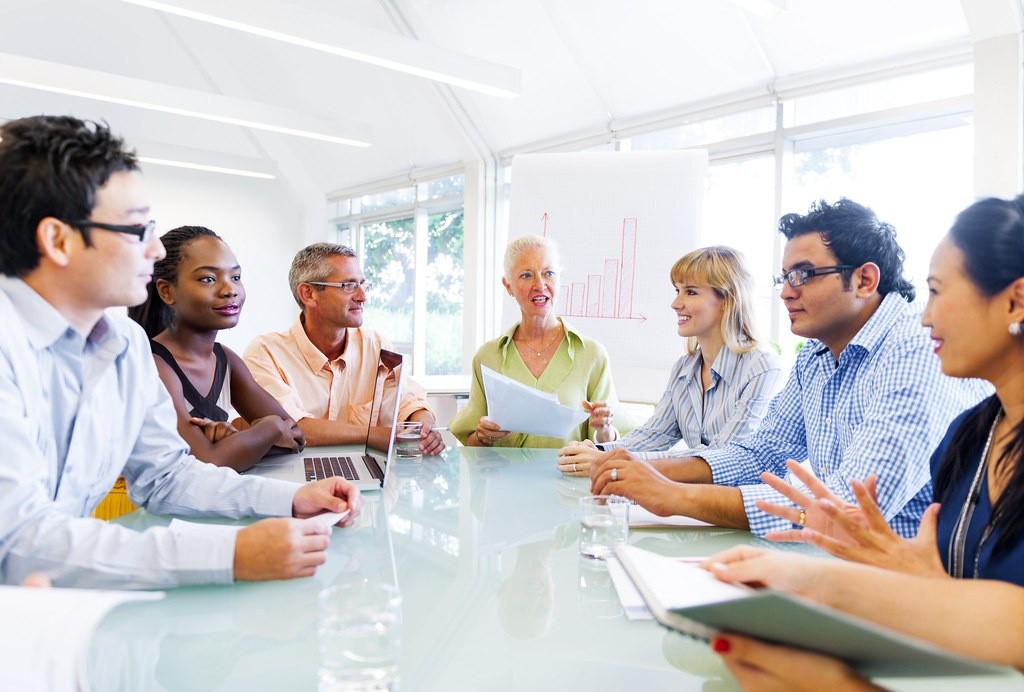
[397,422,423,457]
[580,495,630,558]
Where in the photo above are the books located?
[613,546,1010,680]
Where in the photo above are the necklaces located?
[523,327,560,356]
[953,406,1004,580]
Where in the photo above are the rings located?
[798,510,806,526]
[573,463,577,472]
[611,468,617,481]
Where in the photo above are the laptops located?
[241,348,408,491]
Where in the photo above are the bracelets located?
[475,431,489,446]
[593,426,620,444]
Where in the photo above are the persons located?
[448,235,621,448]
[698,544,1024,692]
[756,194,1023,589]
[590,198,998,540]
[0,114,365,590]
[127,226,306,475]
[245,244,446,458]
[558,245,789,477]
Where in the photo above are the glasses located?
[774,265,851,287]
[308,281,371,293]
[68,220,155,241]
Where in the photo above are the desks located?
[0,446,1024,692]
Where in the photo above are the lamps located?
[121,124,277,180]
[0,11,375,146]
[127,0,529,103]
